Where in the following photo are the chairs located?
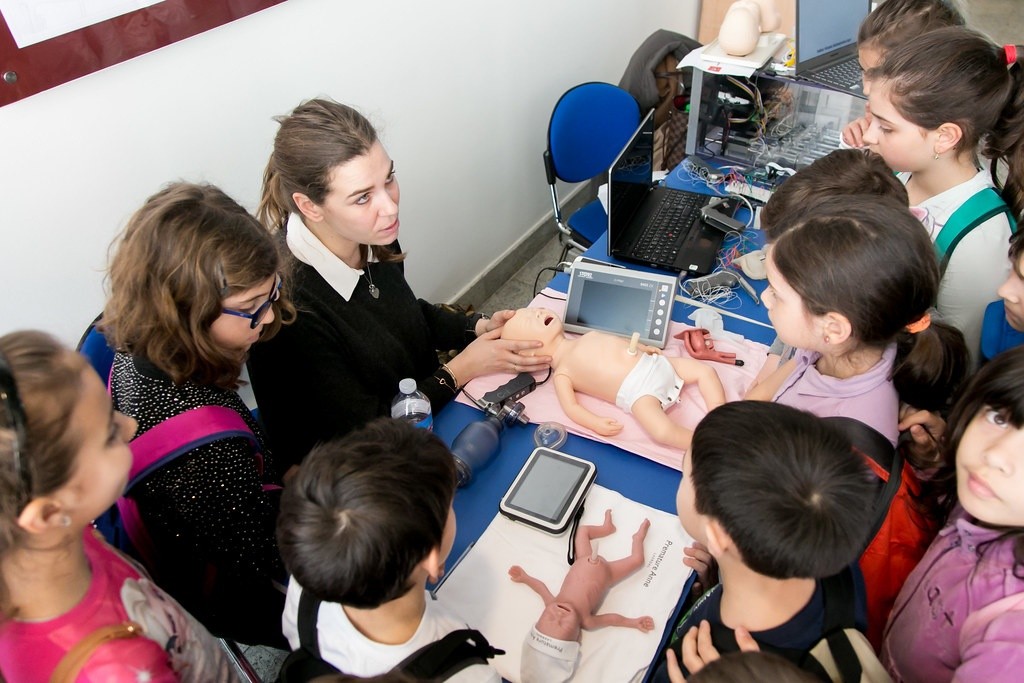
[76,311,128,546]
[542,82,641,264]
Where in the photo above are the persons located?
[719,0,782,55]
[665,620,824,683]
[838,25,1024,378]
[747,147,909,393]
[276,418,501,683]
[508,509,655,683]
[858,0,965,97]
[0,330,240,683]
[501,307,726,450]
[244,98,553,469]
[981,211,1024,359]
[646,400,879,683]
[881,342,1024,683]
[760,195,971,447]
[95,180,291,652]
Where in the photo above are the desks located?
[423,157,834,683]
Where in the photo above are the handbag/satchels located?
[656,70,690,172]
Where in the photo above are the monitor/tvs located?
[564,261,677,350]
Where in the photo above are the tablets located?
[499,447,597,536]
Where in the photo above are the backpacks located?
[74,312,285,617]
[825,416,933,639]
[273,588,505,683]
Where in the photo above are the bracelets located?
[434,376,456,393]
[443,364,458,387]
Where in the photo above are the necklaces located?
[346,261,379,298]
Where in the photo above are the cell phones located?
[568,256,627,269]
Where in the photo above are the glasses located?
[221,274,283,330]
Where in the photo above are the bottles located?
[391,378,433,435]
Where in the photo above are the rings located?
[514,364,516,370]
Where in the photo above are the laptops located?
[795,0,872,100]
[606,109,741,277]
